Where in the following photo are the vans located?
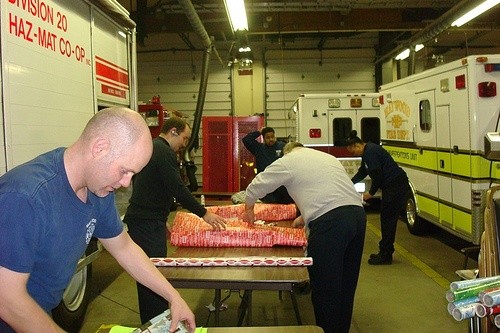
[379,53,500,246]
[286,93,379,209]
[0,1,139,331]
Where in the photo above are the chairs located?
[456,185,500,333]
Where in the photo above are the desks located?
[157,201,311,327]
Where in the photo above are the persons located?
[0,105,196,333]
[241,126,409,333]
[125,118,226,326]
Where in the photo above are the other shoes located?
[368,250,393,265]
